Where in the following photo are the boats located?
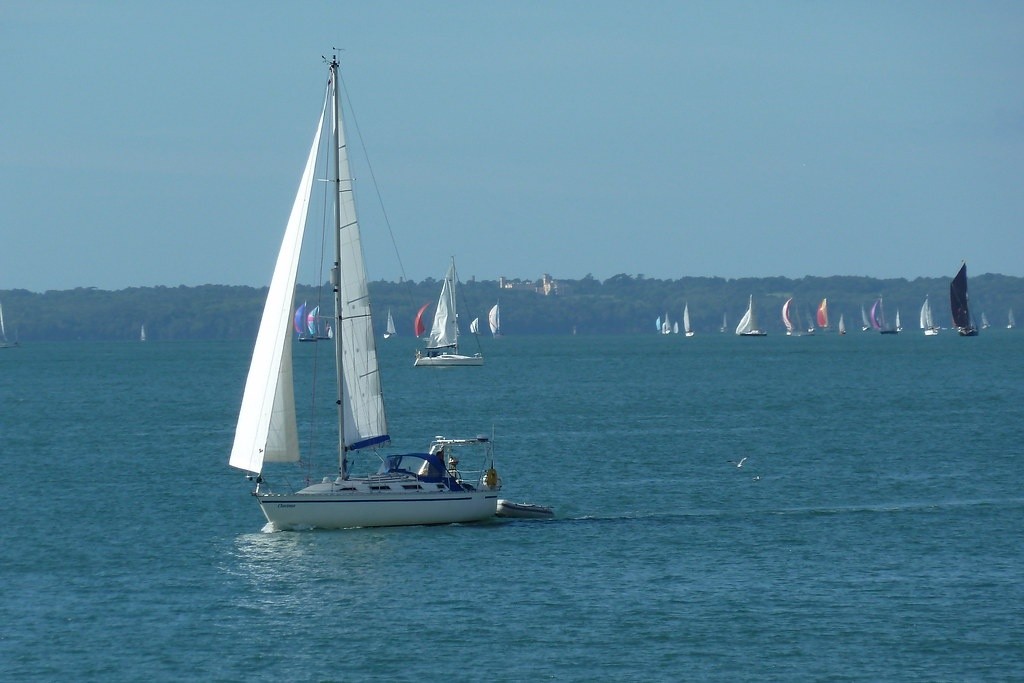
[496,500,552,521]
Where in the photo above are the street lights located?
[861,294,903,335]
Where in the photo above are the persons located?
[436,446,446,466]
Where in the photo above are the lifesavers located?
[488,469,499,487]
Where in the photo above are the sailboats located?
[1006,306,1016,329]
[469,317,482,335]
[840,316,846,336]
[230,50,554,528]
[920,293,940,336]
[817,298,828,331]
[489,299,500,338]
[782,298,816,337]
[294,300,333,342]
[656,313,678,335]
[949,261,979,336]
[141,326,146,343]
[683,303,694,337]
[414,302,431,338]
[383,308,397,338]
[981,313,991,330]
[0,304,20,348]
[721,313,728,332]
[735,294,768,336]
[414,255,484,366]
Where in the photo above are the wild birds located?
[752,472,760,482]
[725,456,750,468]
